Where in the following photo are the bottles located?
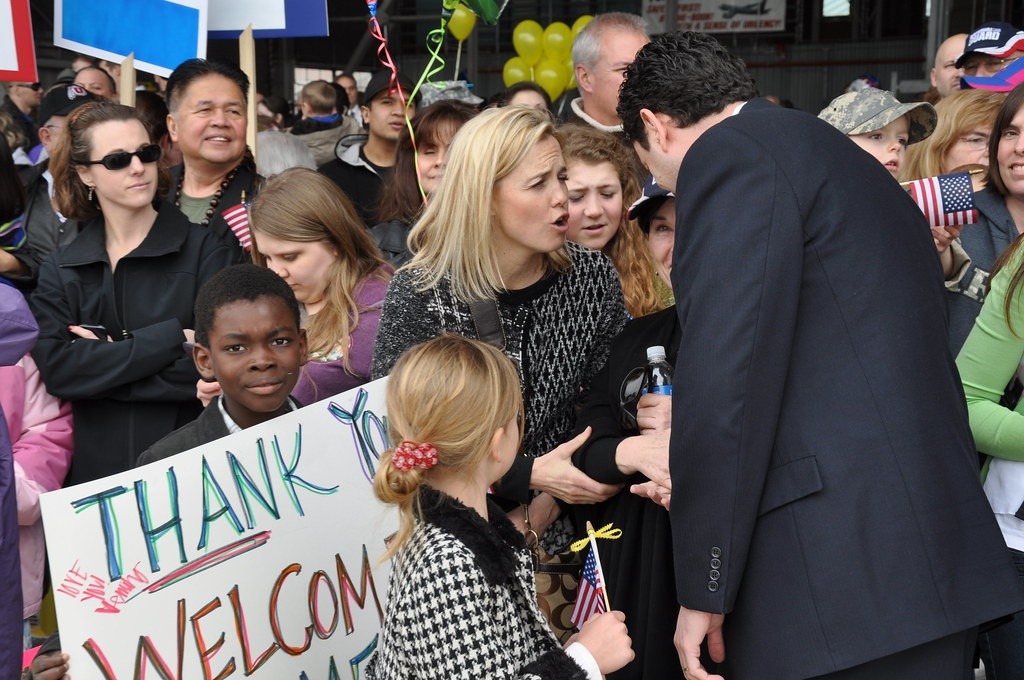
[641,346,674,398]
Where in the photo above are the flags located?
[570,546,605,631]
[223,202,252,249]
[910,172,977,226]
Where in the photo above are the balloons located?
[504,16,595,102]
[448,4,479,41]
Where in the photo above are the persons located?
[617,35,1024,680]
[374,337,635,680]
[30,265,310,680]
[503,80,553,114]
[817,25,1024,576]
[196,166,397,405]
[373,106,627,553]
[558,121,687,680]
[0,55,417,680]
[555,11,649,141]
[30,105,229,484]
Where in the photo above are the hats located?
[848,78,877,93]
[955,20,1024,68]
[629,172,676,235]
[360,71,423,127]
[416,80,483,110]
[37,84,98,128]
[817,87,939,147]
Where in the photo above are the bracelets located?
[524,503,539,569]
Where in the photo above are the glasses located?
[85,143,162,170]
[620,363,649,432]
[961,55,1022,75]
[954,134,991,150]
[9,83,42,92]
